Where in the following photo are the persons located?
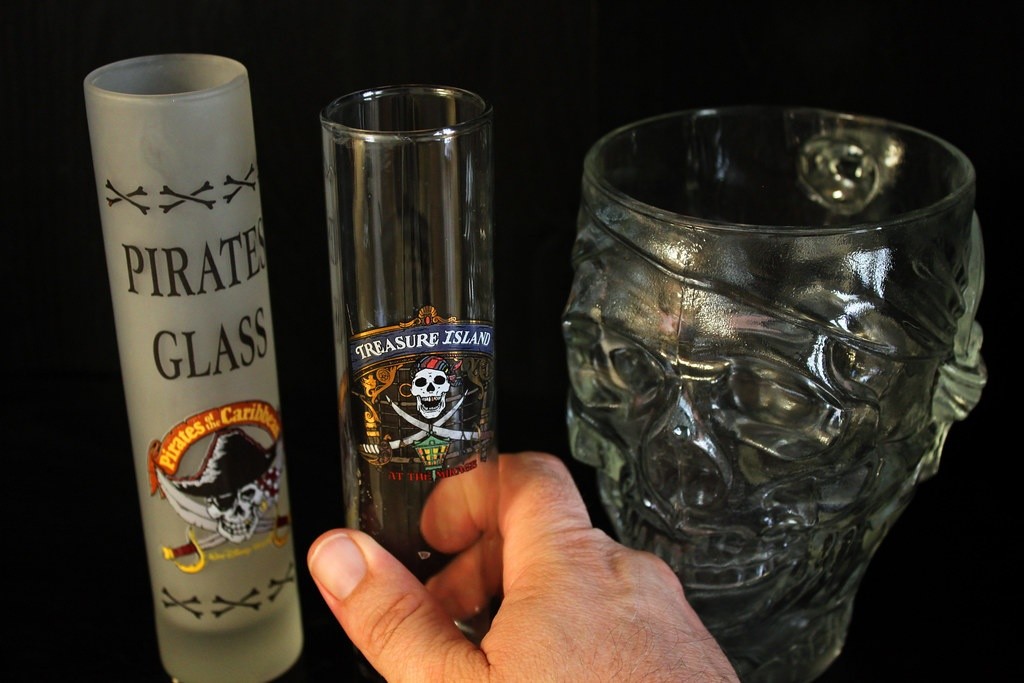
[308,452,742,683]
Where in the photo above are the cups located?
[559,100,989,683]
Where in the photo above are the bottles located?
[322,85,496,644]
[82,53,304,683]
